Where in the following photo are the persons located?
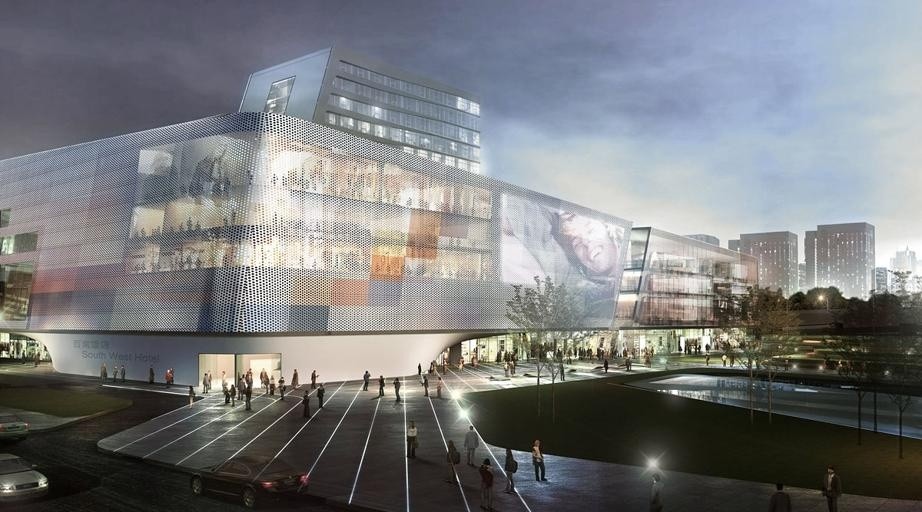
[317,383,326,408]
[443,439,457,482]
[686,340,747,368]
[498,349,518,377]
[260,368,285,400]
[101,363,128,382]
[222,368,253,412]
[302,390,312,417]
[34,352,41,366]
[418,360,447,375]
[464,426,479,465]
[202,370,212,394]
[649,473,665,512]
[820,463,842,512]
[189,385,195,408]
[136,151,181,202]
[291,369,299,389]
[420,376,443,397]
[404,420,419,457]
[393,377,400,402]
[531,439,547,482]
[477,457,493,510]
[458,355,480,371]
[363,371,385,396]
[502,447,516,494]
[560,213,624,288]
[311,370,319,389]
[554,346,654,373]
[21,350,26,364]
[166,368,174,388]
[188,140,232,198]
[768,480,793,512]
[149,365,154,384]
